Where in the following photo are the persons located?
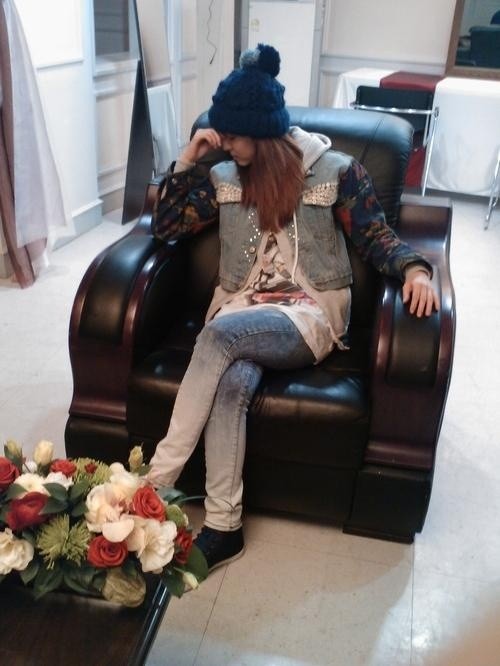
[144,43,440,570]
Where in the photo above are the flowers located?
[0,439,238,608]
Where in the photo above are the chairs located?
[348,86,437,197]
[66,106,457,545]
[455,25,500,69]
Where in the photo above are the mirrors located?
[446,0,499,80]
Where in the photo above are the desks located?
[335,67,500,199]
[0,565,172,666]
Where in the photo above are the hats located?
[208,43,289,135]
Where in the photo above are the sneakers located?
[183,527,245,594]
